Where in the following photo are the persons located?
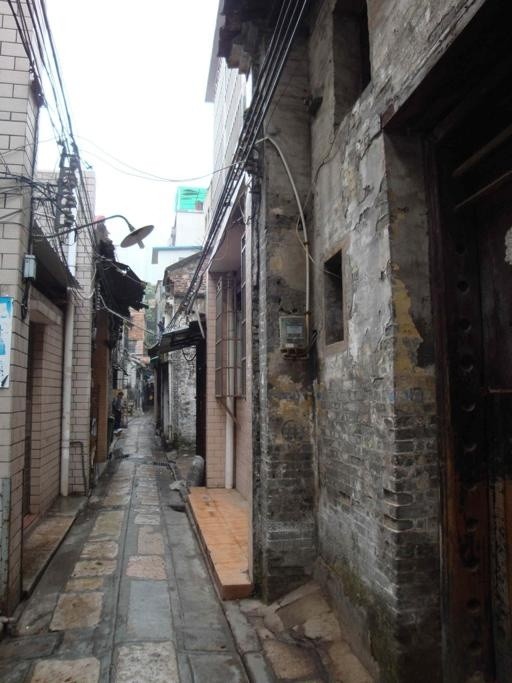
[112,391,124,435]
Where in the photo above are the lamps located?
[31,214,154,248]
